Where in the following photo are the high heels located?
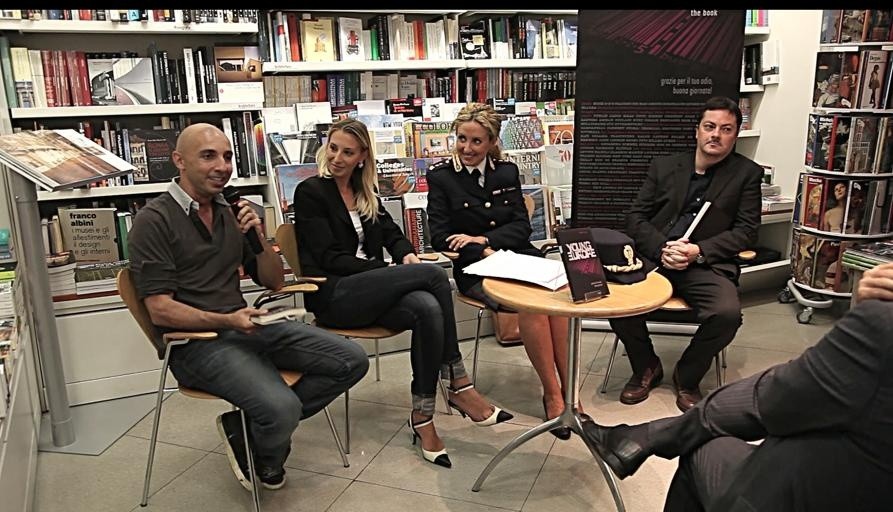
[447,382,514,428]
[407,409,452,468]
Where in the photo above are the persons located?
[126,123,370,490]
[572,260,892,511]
[427,102,590,441]
[602,96,763,416]
[292,116,512,470]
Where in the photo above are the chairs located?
[264,216,451,456]
[111,251,352,512]
[601,246,754,398]
[444,193,536,391]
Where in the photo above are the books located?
[790,6,892,293]
[554,225,610,303]
[0,9,577,327]
[741,9,791,218]
[0,328,28,419]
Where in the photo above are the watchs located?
[695,251,706,265]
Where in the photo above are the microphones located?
[221,185,264,255]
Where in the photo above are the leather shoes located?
[543,395,571,439]
[674,367,703,413]
[577,410,645,480]
[620,359,663,404]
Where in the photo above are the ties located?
[471,169,481,182]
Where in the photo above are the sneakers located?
[217,410,286,490]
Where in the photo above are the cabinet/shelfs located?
[0,324,48,512]
[0,15,297,413]
[259,4,576,363]
[736,24,795,300]
[782,28,892,316]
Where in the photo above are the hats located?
[590,226,646,284]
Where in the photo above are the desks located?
[462,251,675,512]
